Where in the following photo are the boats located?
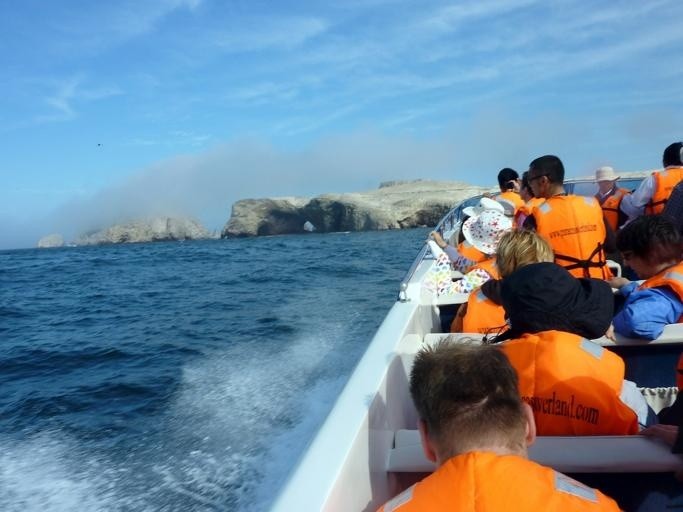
[262,168,683,512]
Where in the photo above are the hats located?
[462,197,514,217]
[592,167,620,183]
[462,210,512,256]
[489,261,615,344]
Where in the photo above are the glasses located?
[526,173,544,184]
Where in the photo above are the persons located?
[411,139,683,341]
[635,356,682,510]
[470,260,659,434]
[371,335,623,511]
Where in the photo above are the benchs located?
[383,258,683,480]
